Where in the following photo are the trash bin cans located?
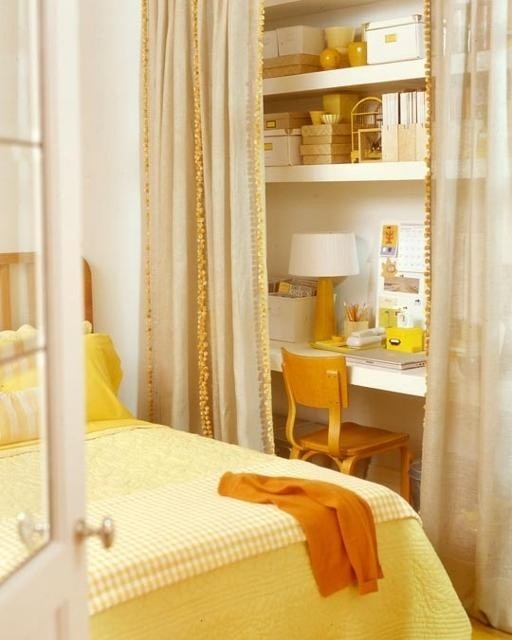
[409,459,422,511]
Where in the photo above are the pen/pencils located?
[345,302,369,321]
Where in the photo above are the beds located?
[0,252,473,640]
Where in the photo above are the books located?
[277,278,319,297]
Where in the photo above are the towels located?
[217,471,385,596]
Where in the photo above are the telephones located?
[346,327,385,347]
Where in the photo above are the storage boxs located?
[268,292,337,342]
[264,14,427,168]
[263,1,510,474]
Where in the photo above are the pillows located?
[0,320,153,451]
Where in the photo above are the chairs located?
[280,346,412,505]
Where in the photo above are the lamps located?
[287,231,360,343]
